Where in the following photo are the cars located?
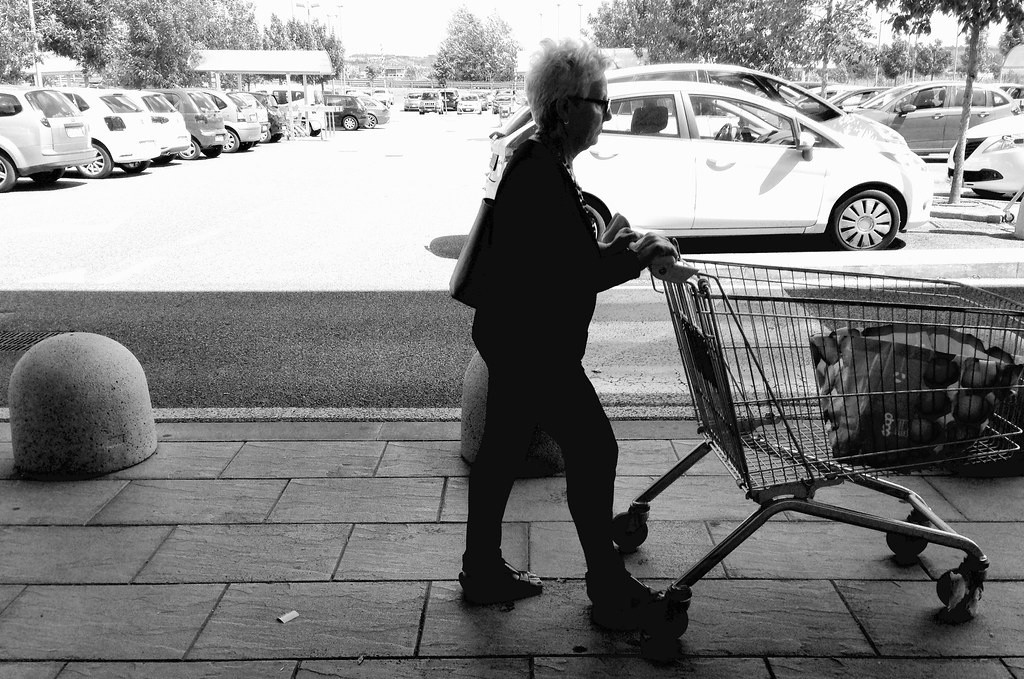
[403,87,529,117]
[106,88,192,165]
[0,82,98,195]
[500,60,908,143]
[139,87,228,162]
[170,82,395,153]
[483,77,933,252]
[947,113,1024,202]
[717,68,1024,154]
[39,85,161,181]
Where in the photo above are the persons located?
[460,37,678,610]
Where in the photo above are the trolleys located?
[610,233,1024,642]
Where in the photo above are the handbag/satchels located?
[821,322,1024,451]
[449,198,494,311]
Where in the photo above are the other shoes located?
[585,570,665,607]
[459,553,544,604]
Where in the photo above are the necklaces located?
[534,128,597,240]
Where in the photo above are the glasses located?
[551,95,611,114]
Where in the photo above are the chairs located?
[631,106,643,135]
[639,106,668,136]
